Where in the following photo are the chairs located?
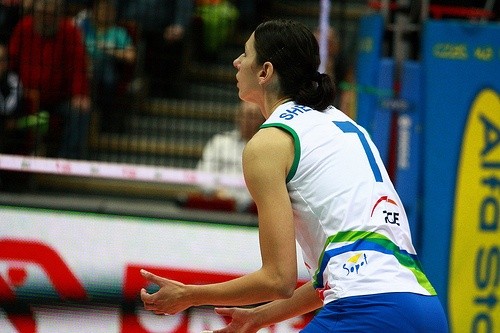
[0,12,197,193]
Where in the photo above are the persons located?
[0,0,266,186]
[139,19,449,333]
